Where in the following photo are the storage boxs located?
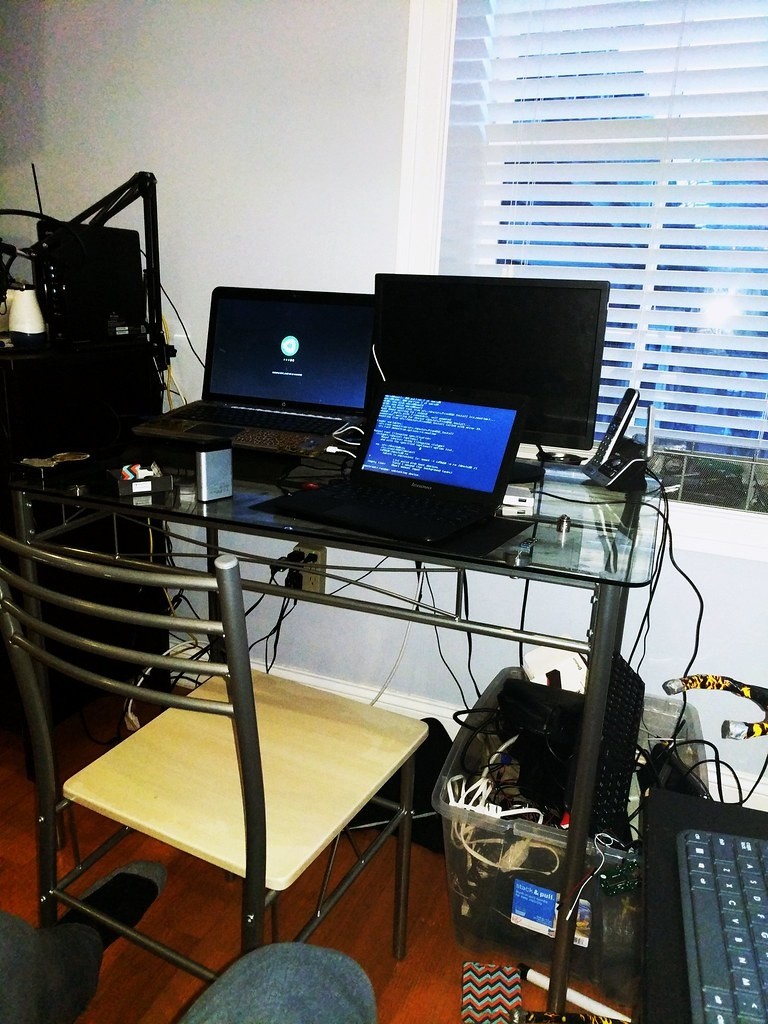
[429,667,710,1006]
[105,466,174,496]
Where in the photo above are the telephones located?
[581,388,654,490]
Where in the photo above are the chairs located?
[0,529,431,986]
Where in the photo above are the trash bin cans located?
[433,667,711,1008]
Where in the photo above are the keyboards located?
[586,653,646,850]
[674,829,768,1024]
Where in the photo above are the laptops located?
[278,386,530,544]
[132,287,374,461]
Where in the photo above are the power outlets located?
[293,543,327,594]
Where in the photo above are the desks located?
[0,332,170,782]
[0,417,663,1023]
[638,786,768,1024]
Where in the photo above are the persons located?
[0,858,378,1024]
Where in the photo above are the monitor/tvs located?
[371,274,610,481]
